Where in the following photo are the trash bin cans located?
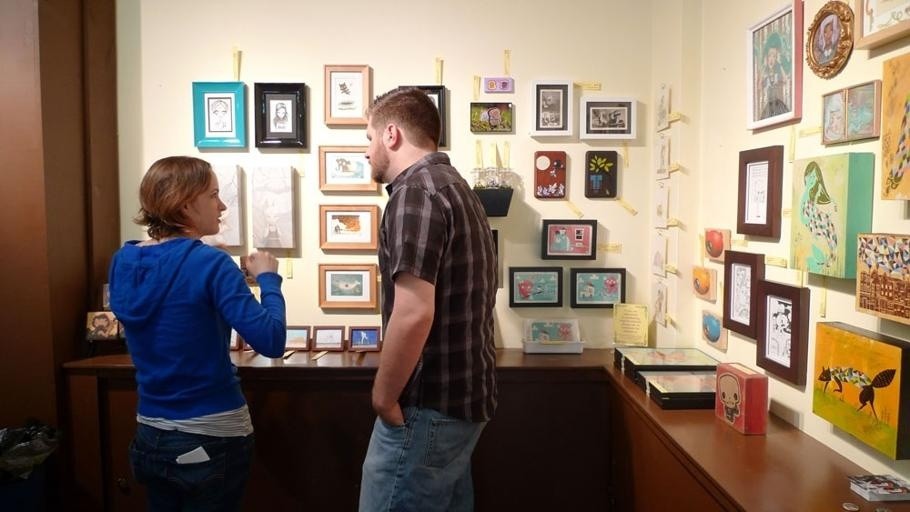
[0,422,71,512]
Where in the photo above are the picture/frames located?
[541,220,598,260]
[192,81,246,149]
[534,150,618,199]
[531,82,574,137]
[570,268,626,308]
[855,233,910,325]
[230,328,254,352]
[723,250,810,388]
[286,325,380,352]
[613,346,721,410]
[319,263,379,313]
[491,228,498,256]
[470,75,516,135]
[204,163,298,251]
[806,1,910,79]
[789,152,876,280]
[612,303,649,347]
[399,85,447,147]
[736,145,785,239]
[813,321,909,461]
[324,64,372,129]
[882,52,910,200]
[253,82,306,149]
[521,318,586,353]
[320,207,380,253]
[821,79,882,145]
[509,266,563,307]
[318,146,382,194]
[747,1,803,131]
[579,99,636,140]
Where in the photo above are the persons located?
[273,101,288,128]
[356,86,500,512]
[818,18,835,64]
[108,158,285,511]
[757,31,788,121]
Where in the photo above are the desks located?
[63,355,910,512]
